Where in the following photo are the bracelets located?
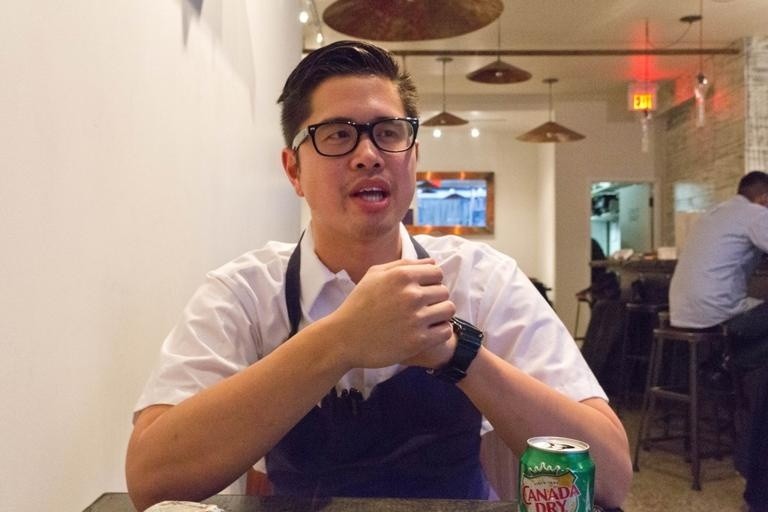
[424,316,485,388]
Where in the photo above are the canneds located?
[517,436,595,512]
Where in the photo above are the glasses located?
[291,116,419,158]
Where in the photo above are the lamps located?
[514,79,587,140]
[418,56,469,128]
[466,15,533,85]
[322,2,505,42]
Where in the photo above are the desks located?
[80,491,621,512]
[588,257,768,276]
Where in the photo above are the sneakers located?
[708,367,751,409]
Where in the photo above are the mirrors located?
[400,170,495,236]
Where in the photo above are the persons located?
[123,39,635,512]
[666,170,768,413]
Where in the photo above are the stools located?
[572,272,747,490]
